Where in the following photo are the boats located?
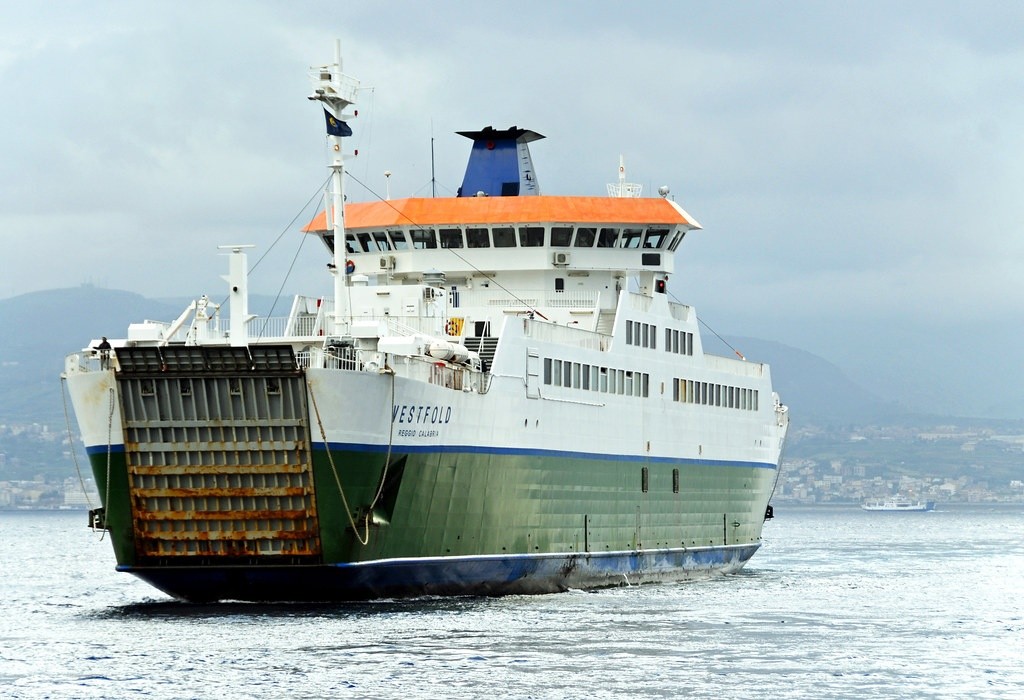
[59,40,790,602]
[860,493,937,512]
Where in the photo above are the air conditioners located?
[555,251,571,264]
[380,256,394,268]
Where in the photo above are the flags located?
[324,108,353,137]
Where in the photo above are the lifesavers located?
[445,321,459,336]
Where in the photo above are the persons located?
[93,336,111,371]
